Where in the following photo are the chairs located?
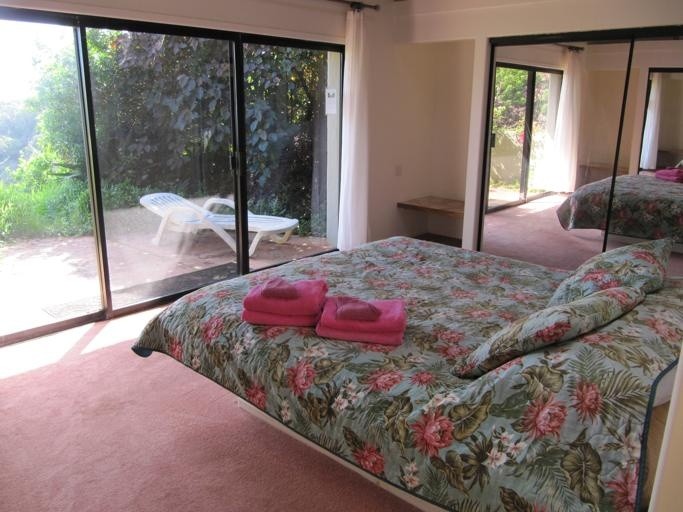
[140,192,298,256]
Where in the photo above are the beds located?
[555,174,683,256]
[129,235,683,512]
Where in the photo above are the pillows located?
[450,237,674,377]
